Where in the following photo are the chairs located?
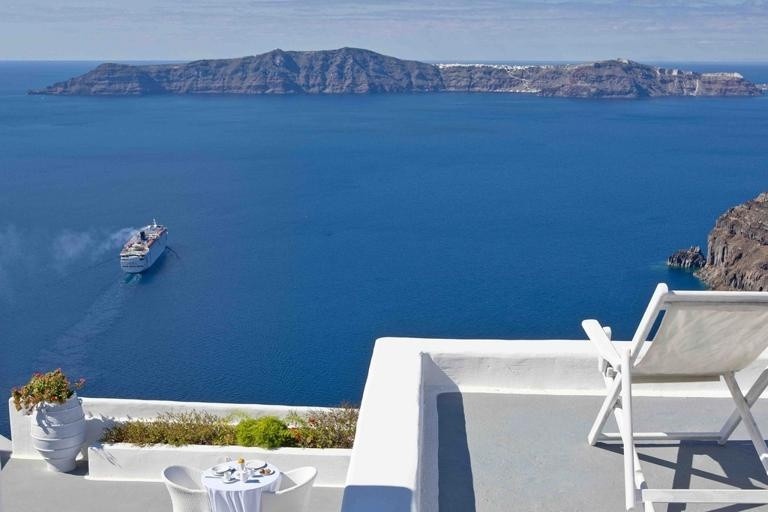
[260,465,319,512]
[163,463,211,512]
[576,280,768,512]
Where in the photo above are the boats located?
[120,225,168,273]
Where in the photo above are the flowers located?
[9,366,86,416]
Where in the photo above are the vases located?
[32,391,86,473]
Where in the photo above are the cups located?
[224,472,231,481]
[249,469,255,477]
[240,472,249,483]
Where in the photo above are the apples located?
[259,468,264,473]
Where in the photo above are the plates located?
[246,460,266,469]
[256,468,273,476]
[211,464,230,473]
